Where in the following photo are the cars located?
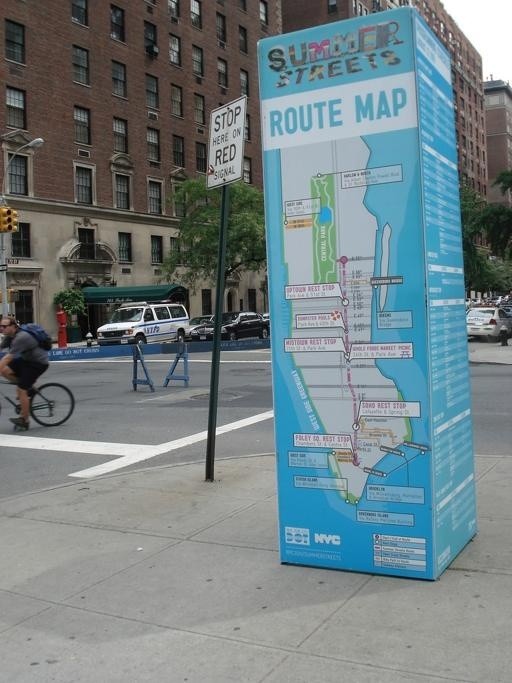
[190,311,270,341]
[465,295,512,346]
[189,315,215,332]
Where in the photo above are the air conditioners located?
[146,45,158,56]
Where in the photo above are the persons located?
[1,315,51,430]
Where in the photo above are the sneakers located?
[15,386,38,401]
[9,417,30,428]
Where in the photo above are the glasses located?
[0,324,13,328]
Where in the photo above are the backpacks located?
[21,324,52,350]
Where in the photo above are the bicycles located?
[1,378,77,432]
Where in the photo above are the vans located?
[96,300,190,347]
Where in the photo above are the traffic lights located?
[1,207,20,234]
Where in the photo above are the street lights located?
[0,137,44,317]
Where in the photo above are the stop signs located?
[205,94,246,191]
